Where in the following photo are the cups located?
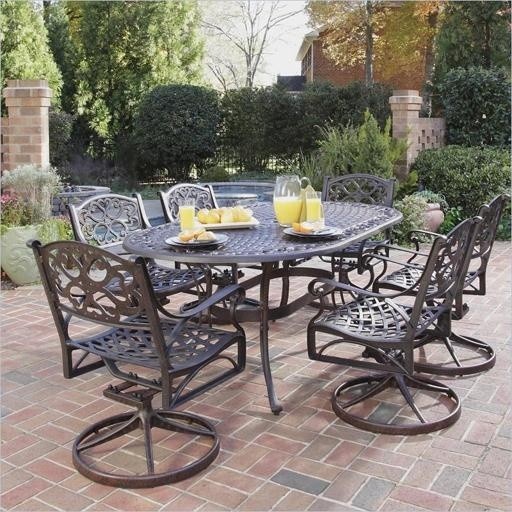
[178,197,195,231]
[273,175,322,227]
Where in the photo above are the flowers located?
[1,162,61,225]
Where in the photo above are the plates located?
[194,216,259,230]
[283,226,342,238]
[164,234,229,247]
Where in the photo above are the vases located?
[0,226,62,286]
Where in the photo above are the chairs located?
[309,175,396,311]
[156,182,259,324]
[66,190,213,327]
[362,193,510,375]
[25,238,247,489]
[307,216,483,436]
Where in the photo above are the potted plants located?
[393,188,449,238]
